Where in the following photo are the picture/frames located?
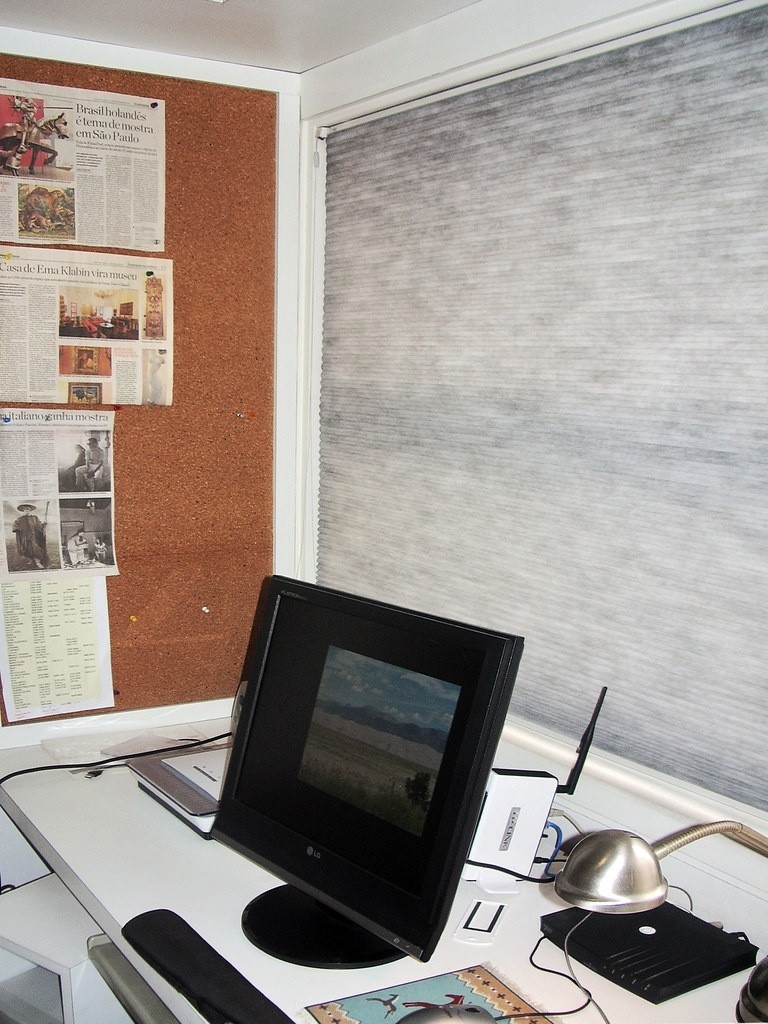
[120,302,133,315]
[72,347,99,375]
[68,381,102,404]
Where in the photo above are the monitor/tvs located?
[209,572,525,970]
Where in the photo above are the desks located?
[59,324,94,338]
[0,714,768,1024]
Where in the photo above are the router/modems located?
[462,685,607,881]
[540,900,760,1004]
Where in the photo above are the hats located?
[17,504,36,512]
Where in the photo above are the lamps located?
[94,288,113,299]
[86,500,95,514]
[113,309,117,316]
[555,821,768,1024]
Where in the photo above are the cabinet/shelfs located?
[0,873,136,1024]
[111,318,137,331]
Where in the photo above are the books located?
[125,742,230,841]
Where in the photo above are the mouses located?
[395,1004,497,1024]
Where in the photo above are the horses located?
[0,112,70,175]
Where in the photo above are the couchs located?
[81,317,105,333]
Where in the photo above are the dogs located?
[0,143,27,176]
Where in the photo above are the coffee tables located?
[98,323,114,337]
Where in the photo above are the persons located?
[12,505,48,568]
[60,437,104,491]
[67,530,107,564]
